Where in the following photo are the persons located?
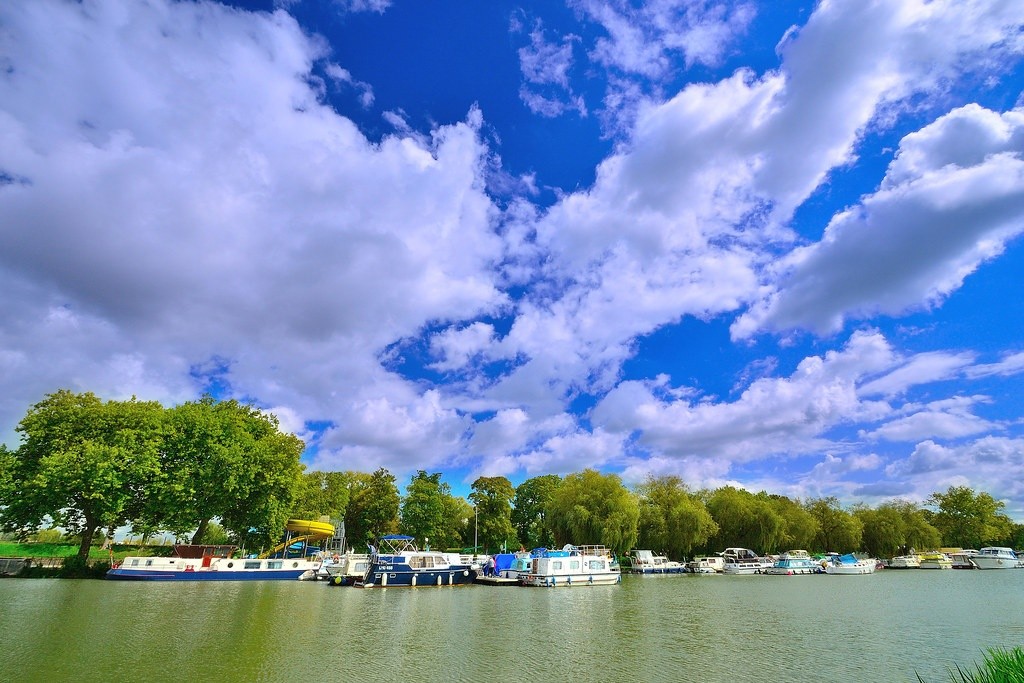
[487,557,496,578]
[365,542,377,558]
[492,556,497,577]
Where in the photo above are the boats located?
[104,557,321,580]
[319,535,480,586]
[511,544,623,588]
[890,547,1023,569]
[692,548,876,574]
[629,549,685,573]
